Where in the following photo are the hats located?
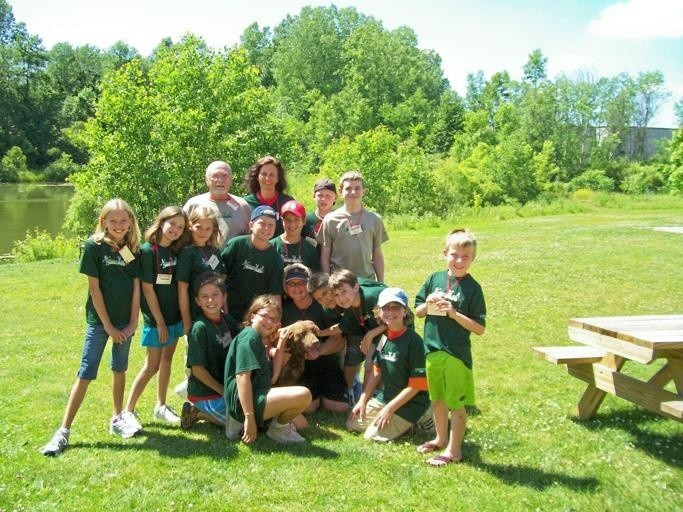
[314,179,337,205]
[250,205,277,221]
[285,264,311,281]
[280,200,306,218]
[377,287,408,310]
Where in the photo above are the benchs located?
[532,347,627,423]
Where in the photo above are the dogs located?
[272,319,320,387]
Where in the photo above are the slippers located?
[427,454,463,467]
[417,440,444,453]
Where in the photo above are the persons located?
[181,271,241,430]
[223,294,312,440]
[300,179,338,239]
[317,172,390,286]
[414,228,486,467]
[175,202,228,399]
[122,205,191,429]
[267,200,322,275]
[220,205,286,323]
[181,161,253,247]
[243,157,296,240]
[344,288,434,440]
[42,197,142,456]
[278,263,413,416]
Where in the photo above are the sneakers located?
[266,416,305,446]
[225,415,243,440]
[43,429,68,457]
[109,381,201,439]
[415,409,436,435]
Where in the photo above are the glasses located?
[256,312,280,324]
[287,281,306,286]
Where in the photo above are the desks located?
[572,317,683,423]
